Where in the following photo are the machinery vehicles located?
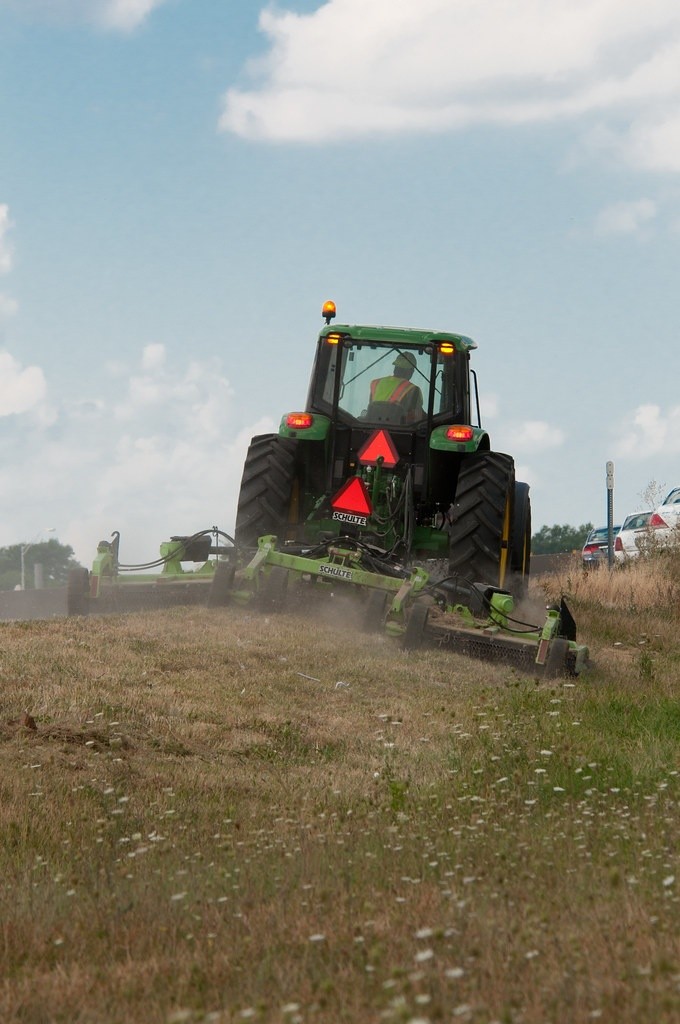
[65,299,590,680]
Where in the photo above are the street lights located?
[20,527,55,590]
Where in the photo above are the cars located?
[582,488,680,568]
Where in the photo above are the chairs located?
[366,402,406,425]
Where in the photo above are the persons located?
[368,352,423,425]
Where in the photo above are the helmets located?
[392,352,416,369]
